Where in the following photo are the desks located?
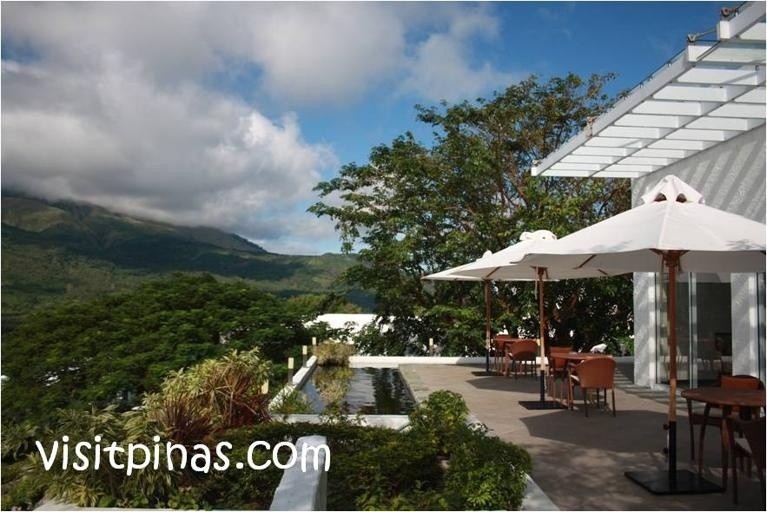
[682,388,765,490]
[551,352,613,408]
[491,339,540,375]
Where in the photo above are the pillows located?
[720,375,760,412]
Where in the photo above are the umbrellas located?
[504,173,766,480]
[417,248,560,370]
[443,228,633,404]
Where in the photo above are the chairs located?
[568,357,616,417]
[501,341,538,377]
[494,335,513,371]
[547,345,578,407]
[698,375,766,478]
[722,416,766,506]
